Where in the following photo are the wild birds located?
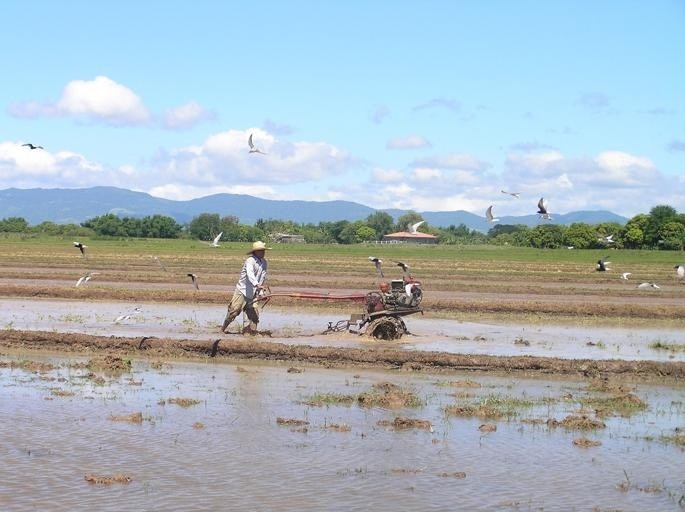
[588,232,685,289]
[407,220,425,236]
[21,143,44,149]
[75,271,103,288]
[368,255,409,278]
[73,240,89,254]
[483,189,552,223]
[198,229,225,248]
[187,273,200,291]
[205,339,222,358]
[248,134,267,153]
[404,279,422,297]
[138,335,152,350]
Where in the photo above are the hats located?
[244,241,273,255]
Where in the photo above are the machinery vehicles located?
[247,275,424,342]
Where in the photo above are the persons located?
[218,239,272,335]
